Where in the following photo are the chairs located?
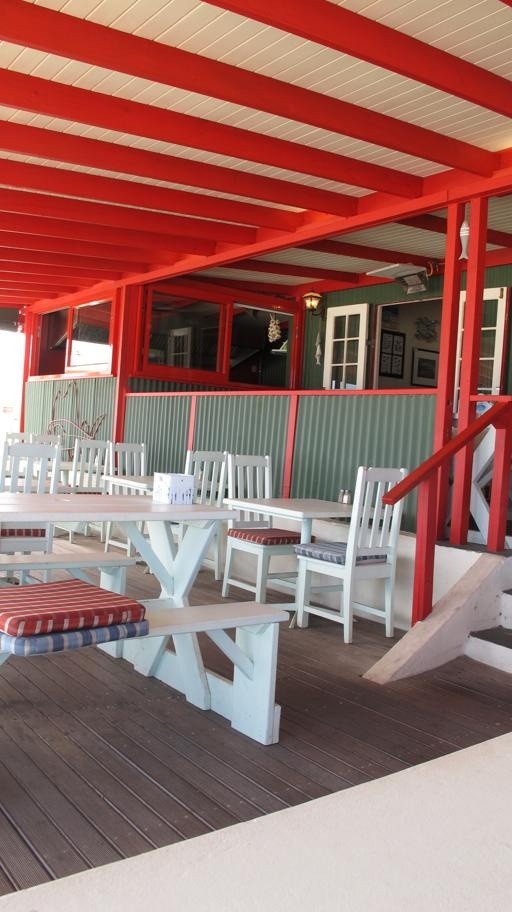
[150,450,317,602]
[0,435,149,585]
[294,465,408,645]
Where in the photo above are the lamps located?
[394,260,436,296]
[303,287,325,321]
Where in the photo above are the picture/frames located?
[411,347,439,388]
[379,329,406,378]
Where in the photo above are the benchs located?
[2,601,289,749]
